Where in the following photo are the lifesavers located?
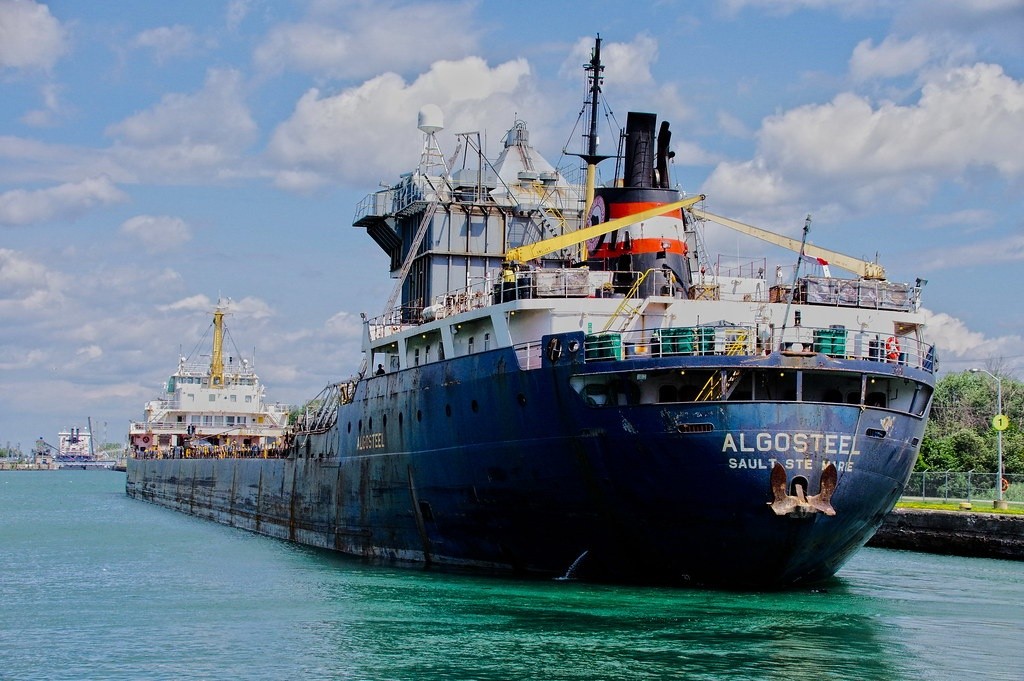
[886,337,900,359]
[1002,478,1008,491]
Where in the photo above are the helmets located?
[501,260,510,264]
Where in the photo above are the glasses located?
[447,298,452,300]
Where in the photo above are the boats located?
[52,426,116,470]
[125,33,939,593]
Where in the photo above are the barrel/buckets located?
[899,352,908,366]
[503,282,516,302]
[491,283,501,305]
[869,340,884,363]
[595,289,610,298]
[517,278,530,299]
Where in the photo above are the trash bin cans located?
[600,333,621,362]
[676,327,696,355]
[697,328,715,356]
[832,328,848,354]
[656,329,676,356]
[817,329,836,353]
[585,334,601,362]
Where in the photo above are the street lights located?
[969,369,1002,500]
